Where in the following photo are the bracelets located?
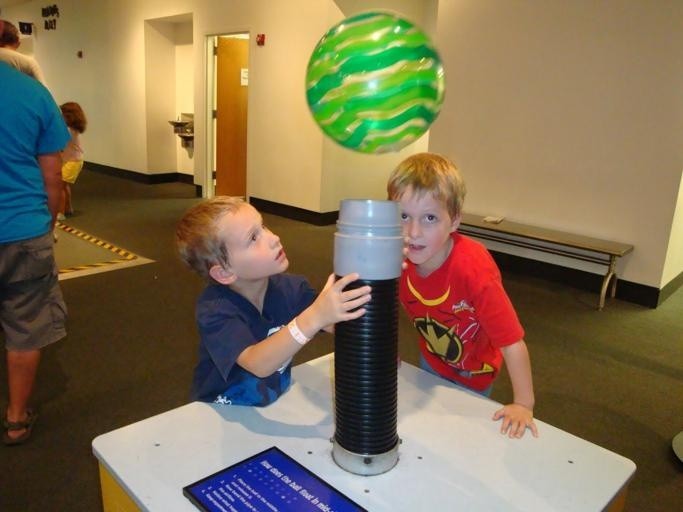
[288,318,311,349]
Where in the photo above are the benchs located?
[456,212,634,312]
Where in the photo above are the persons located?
[54,102,87,219]
[0,19,47,89]
[387,153,539,438]
[0,61,70,444]
[175,195,372,407]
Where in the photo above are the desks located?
[89,352,637,511]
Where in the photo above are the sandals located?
[2,405,38,443]
